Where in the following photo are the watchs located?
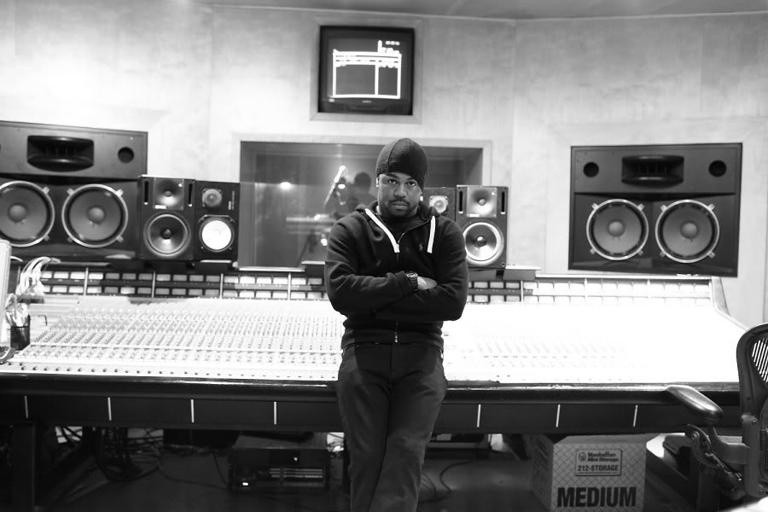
[406,271,418,291]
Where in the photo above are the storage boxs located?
[530,433,661,512]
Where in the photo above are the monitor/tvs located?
[318,25,415,115]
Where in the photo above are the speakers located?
[135,174,241,269]
[568,142,743,278]
[0,120,148,263]
[422,183,508,270]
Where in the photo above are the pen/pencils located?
[5,311,30,347]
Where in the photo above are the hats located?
[376,138,428,191]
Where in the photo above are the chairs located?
[663,323,768,512]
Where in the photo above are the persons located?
[347,170,377,212]
[324,135,468,512]
